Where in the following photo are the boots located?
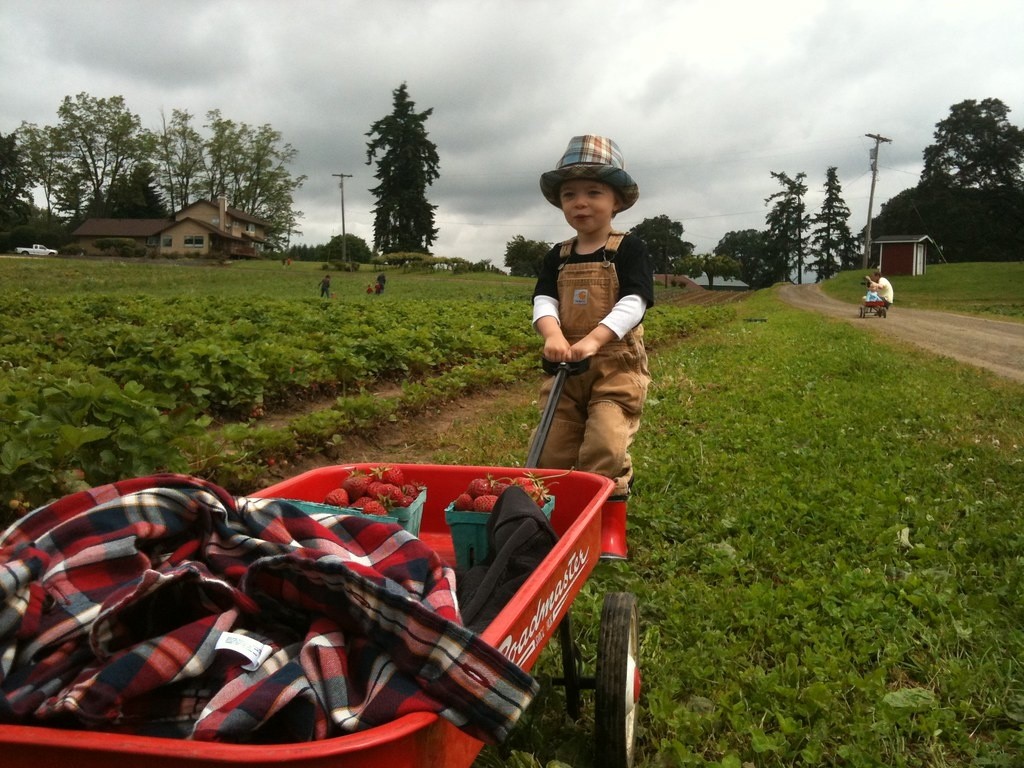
[601,499,629,559]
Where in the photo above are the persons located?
[531,134,654,561]
[281,257,292,268]
[367,272,387,294]
[865,271,893,316]
[318,275,330,297]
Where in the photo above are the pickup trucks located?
[15,244,58,256]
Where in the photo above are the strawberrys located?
[323,464,423,516]
[452,468,551,512]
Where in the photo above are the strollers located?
[858,281,888,320]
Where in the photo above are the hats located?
[540,135,639,212]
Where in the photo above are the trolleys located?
[0,355,644,767]
